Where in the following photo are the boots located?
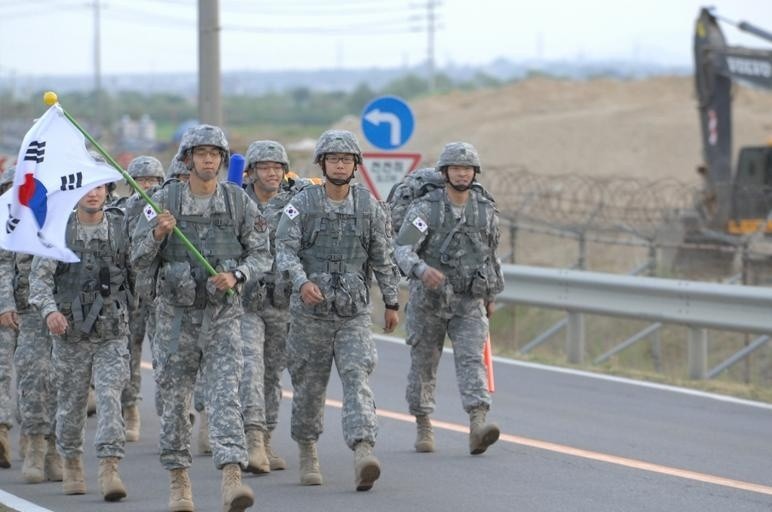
[221,461,255,511]
[98,456,129,503]
[414,413,435,453]
[299,439,324,486]
[246,430,270,476]
[468,405,500,455]
[265,436,287,471]
[21,436,47,483]
[0,425,13,467]
[354,443,382,492]
[124,404,143,442]
[48,438,66,483]
[62,456,87,496]
[199,415,213,452]
[89,387,98,414]
[168,470,194,512]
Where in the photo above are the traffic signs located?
[362,97,416,151]
[357,151,421,205]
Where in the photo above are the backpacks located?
[386,168,496,277]
[121,179,245,256]
[264,178,371,282]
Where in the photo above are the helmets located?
[244,140,289,173]
[90,147,106,163]
[313,130,364,165]
[169,150,191,177]
[1,164,17,183]
[438,142,481,173]
[124,157,166,186]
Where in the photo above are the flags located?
[0,92,238,297]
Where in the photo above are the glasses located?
[136,175,160,187]
[325,156,356,164]
[255,164,284,170]
[194,149,220,159]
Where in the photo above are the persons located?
[242,139,298,474]
[392,141,506,455]
[0,167,15,195]
[130,125,274,512]
[28,151,131,502]
[0,249,64,482]
[122,155,165,441]
[0,167,16,469]
[275,128,399,492]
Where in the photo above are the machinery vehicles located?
[670,8,772,281]
